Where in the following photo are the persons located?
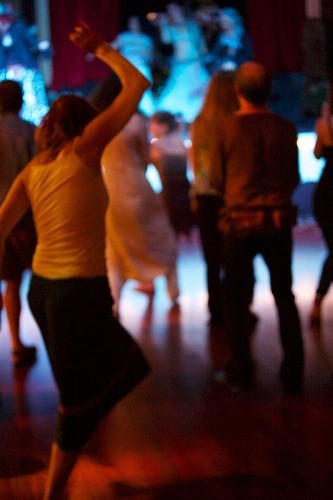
[309,91,333,322]
[0,80,39,362]
[0,17,152,500]
[190,60,306,395]
[85,72,198,316]
